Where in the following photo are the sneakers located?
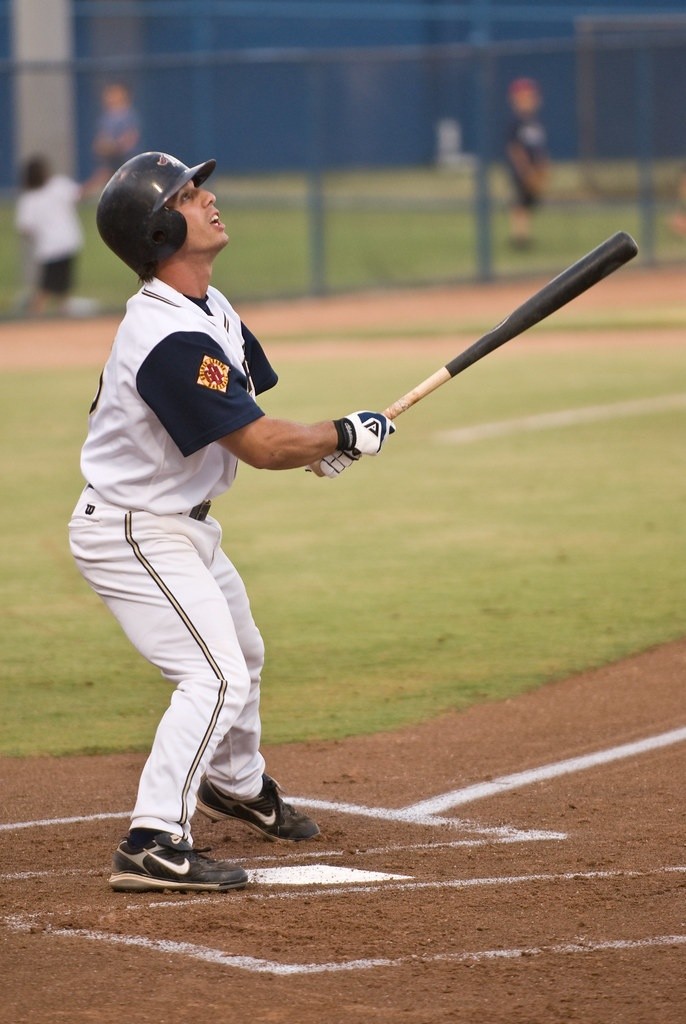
[196,770,320,846]
[108,832,248,892]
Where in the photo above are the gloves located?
[333,410,396,457]
[306,449,361,479]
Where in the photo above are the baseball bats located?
[308,229,639,478]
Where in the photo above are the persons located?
[504,77,550,249]
[13,154,109,318]
[91,80,144,171]
[69,151,396,893]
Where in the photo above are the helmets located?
[95,151,217,279]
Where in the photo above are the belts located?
[189,499,211,522]
